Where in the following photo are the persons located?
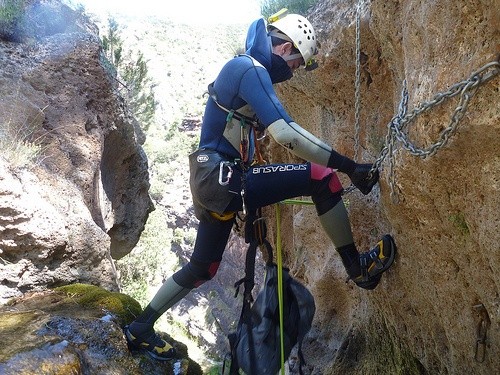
[124,7,395,360]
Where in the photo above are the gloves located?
[244,215,268,244]
[338,160,379,195]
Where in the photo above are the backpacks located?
[228,243,316,375]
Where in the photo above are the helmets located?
[266,8,318,71]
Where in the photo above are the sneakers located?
[123,323,176,360]
[350,233,396,291]
